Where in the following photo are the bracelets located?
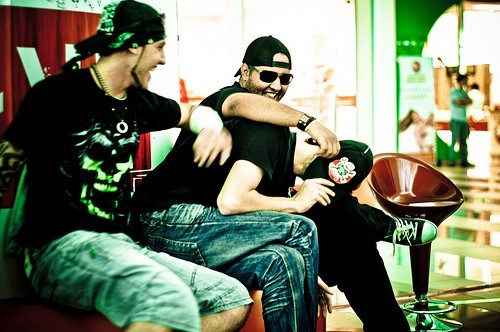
[297,113,316,132]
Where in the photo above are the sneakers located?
[383,216,439,246]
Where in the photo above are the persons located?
[398,110,434,153]
[128,35,484,332]
[0,0,254,332]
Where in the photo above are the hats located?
[73,0,165,56]
[233,36,291,78]
[303,139,374,193]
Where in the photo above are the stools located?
[366,153,465,332]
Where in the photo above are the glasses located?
[249,64,293,85]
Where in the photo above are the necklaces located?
[91,62,128,134]
